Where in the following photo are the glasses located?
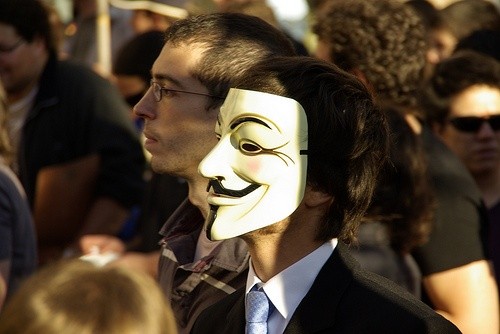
[147,79,225,102]
[125,92,144,107]
[447,115,500,133]
[0,38,26,54]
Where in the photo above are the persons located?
[133,12,297,334]
[198,54,461,334]
[0,0,500,334]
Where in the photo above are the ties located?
[246,286,276,334]
